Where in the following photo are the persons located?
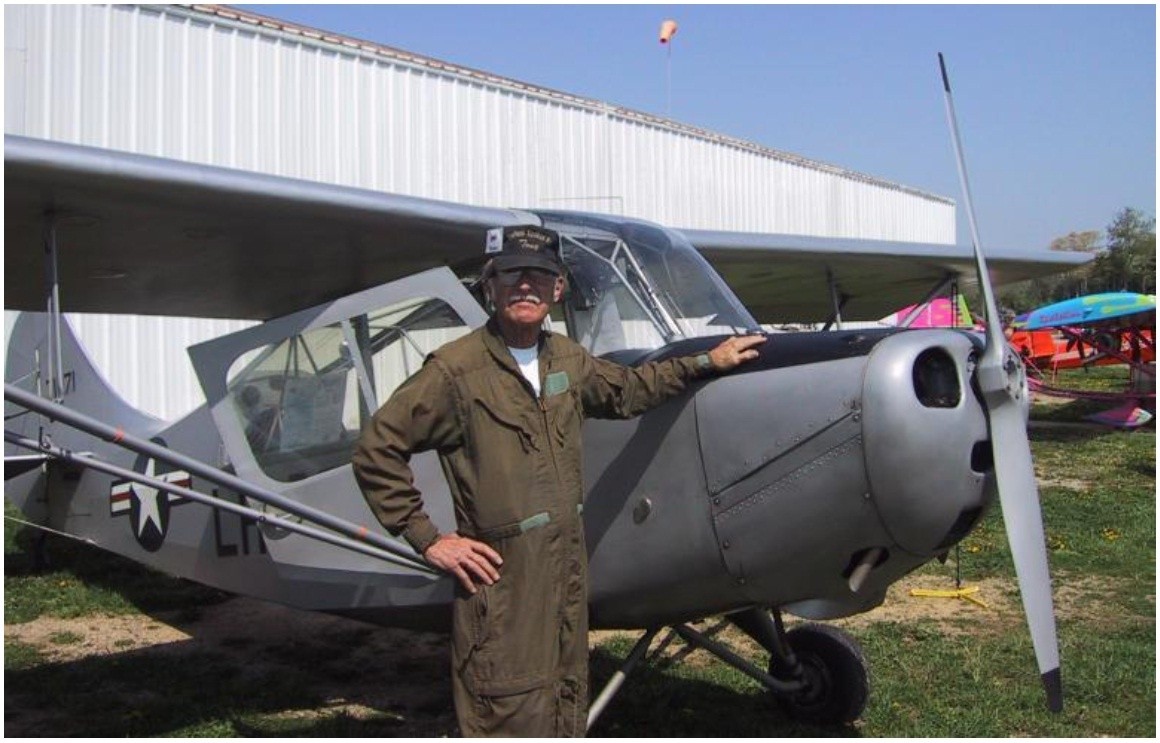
[348,223,771,738]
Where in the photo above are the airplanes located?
[950,292,1156,427]
[5,50,1062,738]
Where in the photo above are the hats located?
[482,224,563,280]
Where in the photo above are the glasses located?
[488,268,556,286]
